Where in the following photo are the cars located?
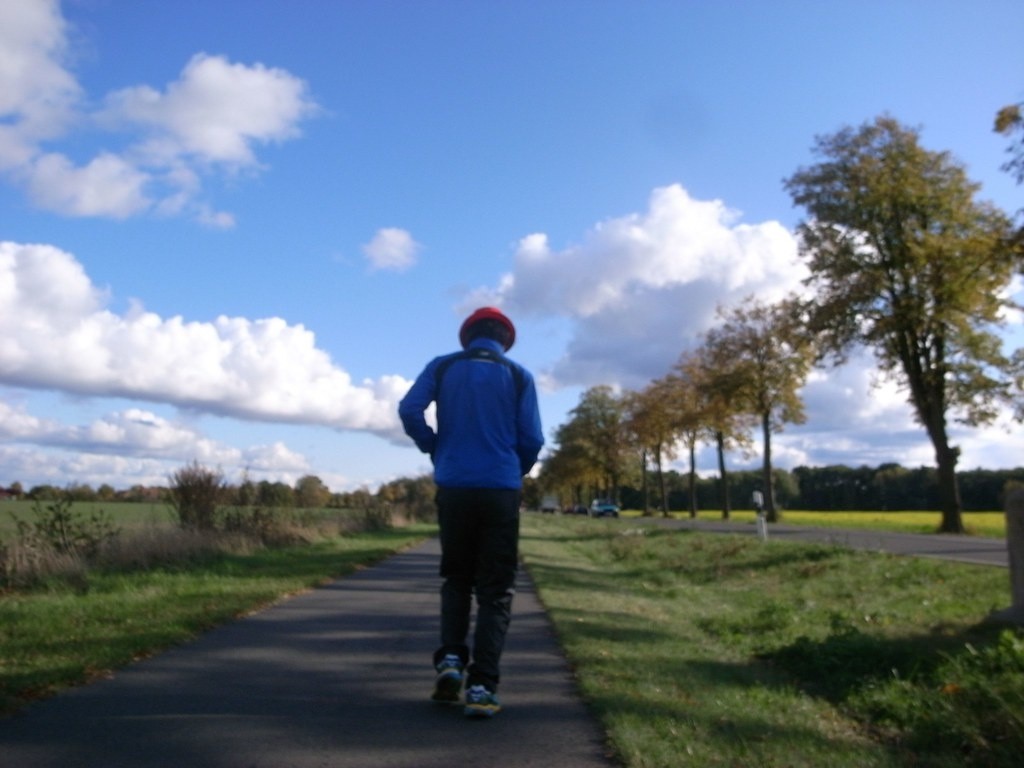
[591,498,621,518]
[542,502,562,514]
[566,505,588,515]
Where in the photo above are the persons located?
[399,307,544,714]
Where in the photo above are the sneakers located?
[430,654,462,701]
[465,685,501,712]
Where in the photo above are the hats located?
[459,307,515,353]
